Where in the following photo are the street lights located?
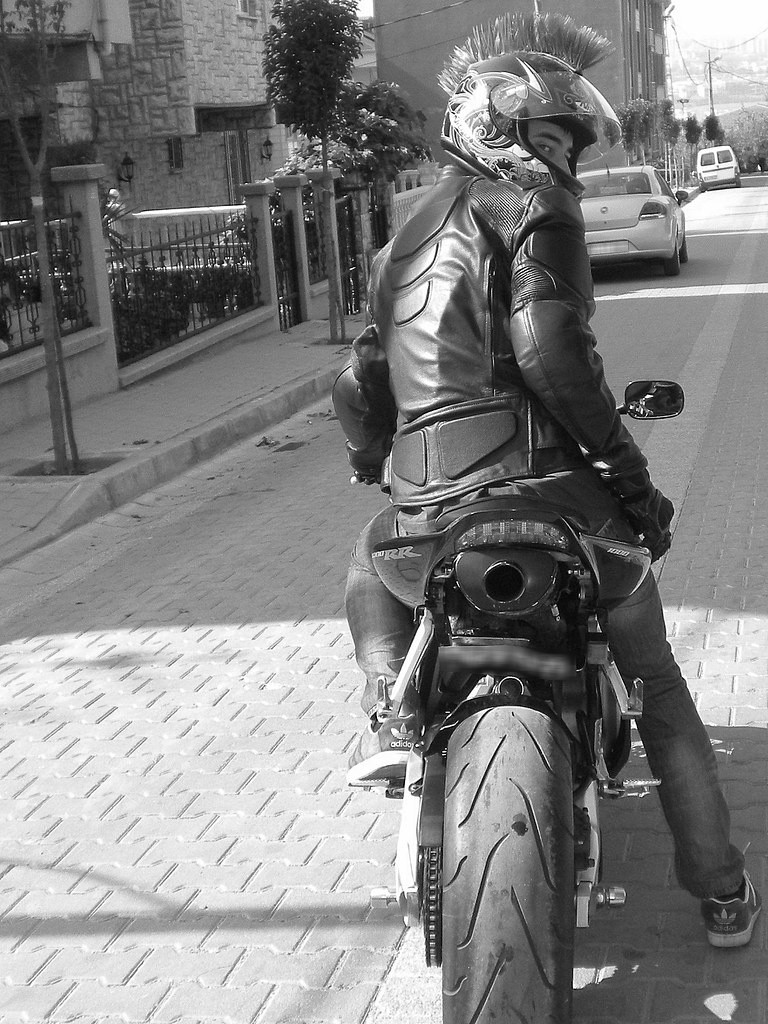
[676,98,689,118]
[706,55,722,114]
[665,2,677,102]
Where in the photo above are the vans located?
[697,145,742,193]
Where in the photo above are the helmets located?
[440,50,597,203]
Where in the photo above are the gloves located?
[619,469,675,563]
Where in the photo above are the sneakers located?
[347,714,419,792]
[701,868,762,947]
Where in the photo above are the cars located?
[578,161,690,272]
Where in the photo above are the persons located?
[331,50,763,948]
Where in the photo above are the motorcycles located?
[359,370,684,1023]
